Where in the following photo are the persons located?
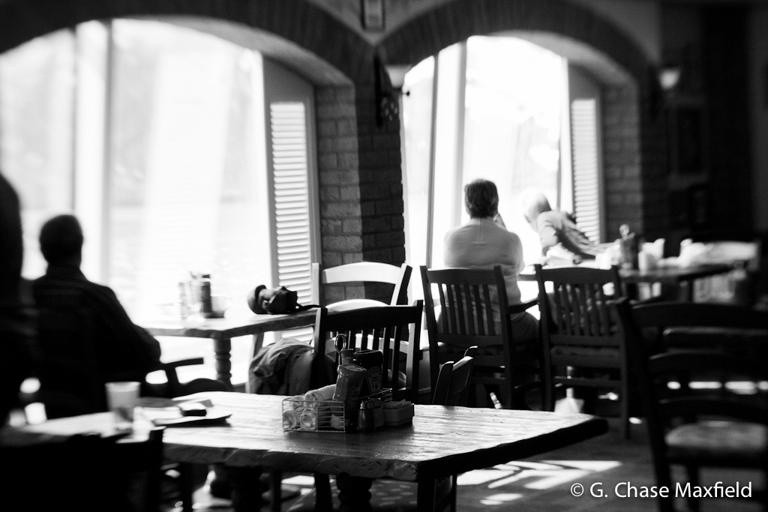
[0,175,160,511]
[521,192,599,260]
[22,214,161,421]
[444,177,543,409]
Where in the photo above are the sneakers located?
[211,477,270,497]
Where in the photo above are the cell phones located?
[181,403,206,415]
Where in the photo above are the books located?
[134,405,233,427]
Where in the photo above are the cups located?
[105,381,141,433]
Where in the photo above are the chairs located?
[310,237,766,511]
[0,426,164,511]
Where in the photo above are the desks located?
[19,303,610,512]
